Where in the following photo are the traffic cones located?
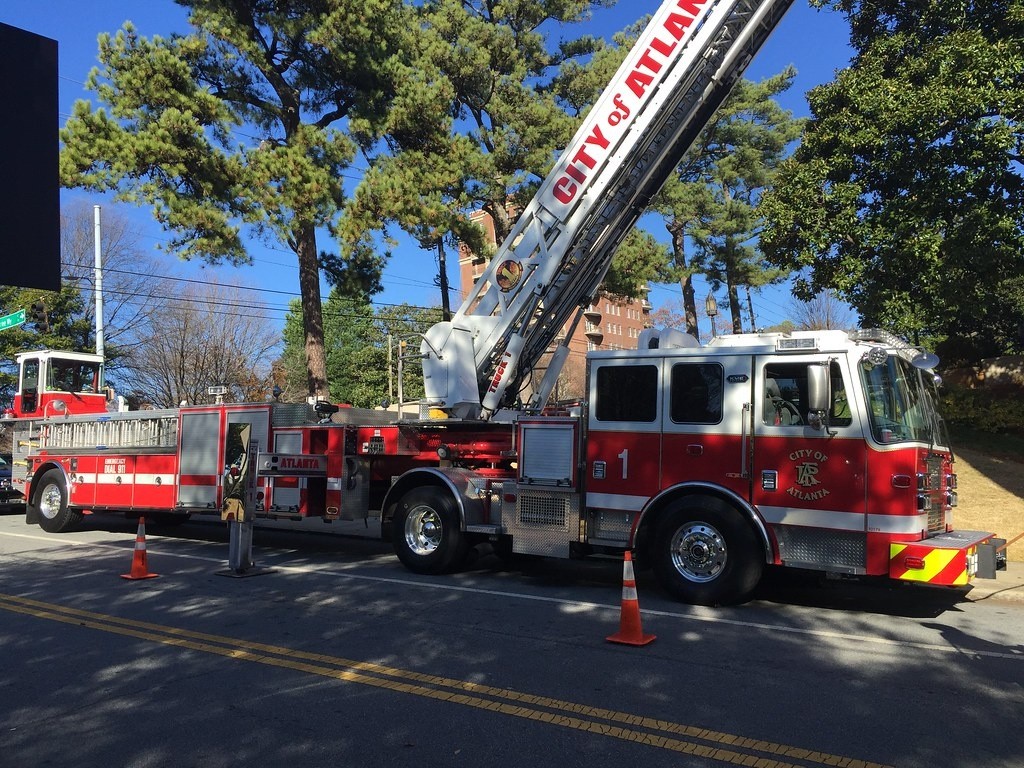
[119,516,159,580]
[605,550,657,646]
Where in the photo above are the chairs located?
[767,379,782,426]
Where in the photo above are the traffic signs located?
[0,308,26,332]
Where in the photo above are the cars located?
[0,456,25,503]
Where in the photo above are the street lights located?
[704,290,719,338]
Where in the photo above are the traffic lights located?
[30,301,50,335]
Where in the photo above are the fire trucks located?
[0,0,1012,601]
